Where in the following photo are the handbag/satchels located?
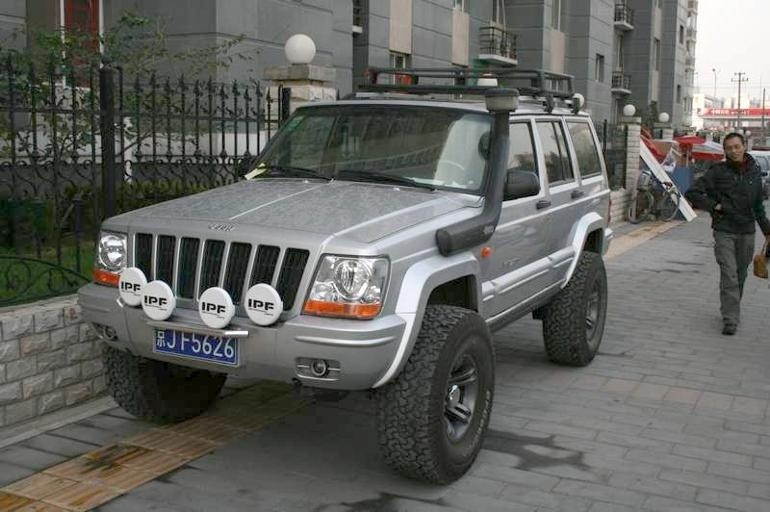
[752,234,770,280]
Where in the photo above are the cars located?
[748,148,770,201]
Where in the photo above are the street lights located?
[710,66,718,134]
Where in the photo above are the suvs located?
[76,62,613,488]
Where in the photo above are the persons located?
[684,133,770,337]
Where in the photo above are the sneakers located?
[722,322,738,336]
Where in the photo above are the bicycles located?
[625,170,682,223]
